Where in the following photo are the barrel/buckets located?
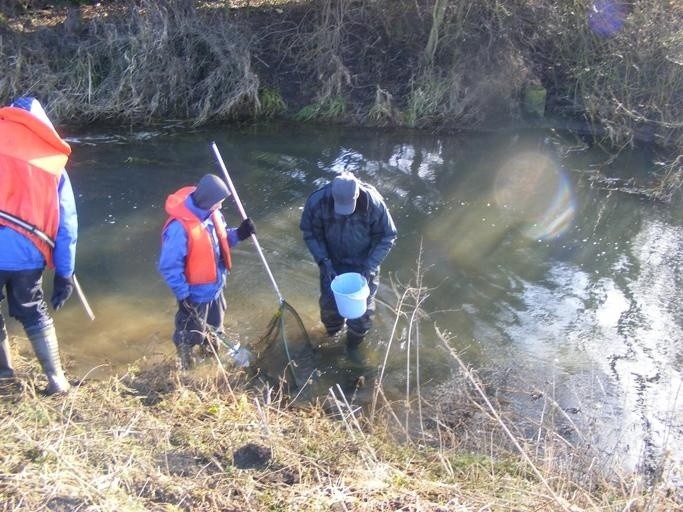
[330,272,370,319]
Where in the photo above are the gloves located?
[237,218,257,241]
[322,258,337,282]
[50,276,74,311]
[179,300,193,313]
[361,261,379,286]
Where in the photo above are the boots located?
[0,317,13,378]
[23,318,70,392]
[323,321,343,337]
[346,326,369,364]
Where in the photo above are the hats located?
[330,176,359,215]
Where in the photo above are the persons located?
[299,172,397,351]
[0,96,77,395]
[159,174,257,358]
